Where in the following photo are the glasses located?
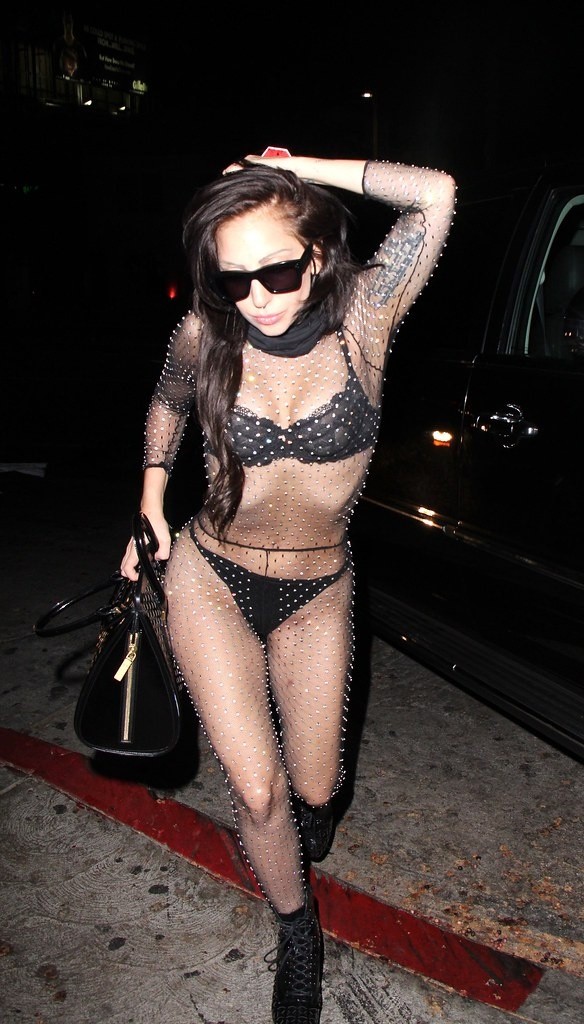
[208,243,315,304]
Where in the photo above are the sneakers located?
[263,897,324,1023]
[299,809,337,864]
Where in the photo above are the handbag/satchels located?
[31,512,200,759]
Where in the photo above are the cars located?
[170,173,584,767]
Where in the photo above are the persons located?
[121,155,459,1024]
